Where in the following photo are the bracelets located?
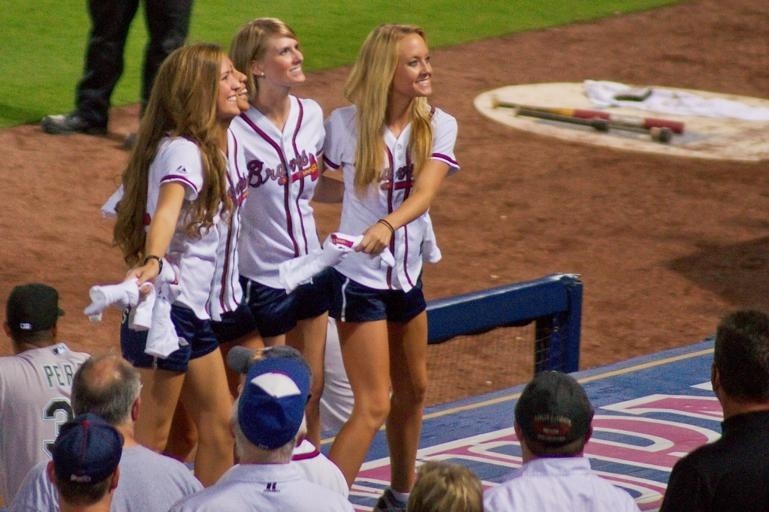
[375,217,403,238]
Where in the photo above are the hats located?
[515,371,595,442]
[238,357,310,449]
[51,413,122,482]
[7,283,66,333]
[226,345,304,376]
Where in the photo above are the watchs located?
[140,250,168,279]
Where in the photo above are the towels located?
[84,257,180,359]
[278,232,396,295]
[101,183,124,214]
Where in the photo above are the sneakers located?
[371,490,408,512]
[42,112,105,136]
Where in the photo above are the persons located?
[231,19,333,458]
[45,413,126,511]
[323,26,460,512]
[224,345,353,499]
[10,351,202,511]
[89,44,236,479]
[486,370,645,512]
[656,310,769,512]
[0,283,96,512]
[41,1,193,138]
[167,358,355,512]
[167,43,248,479]
[402,460,487,512]
